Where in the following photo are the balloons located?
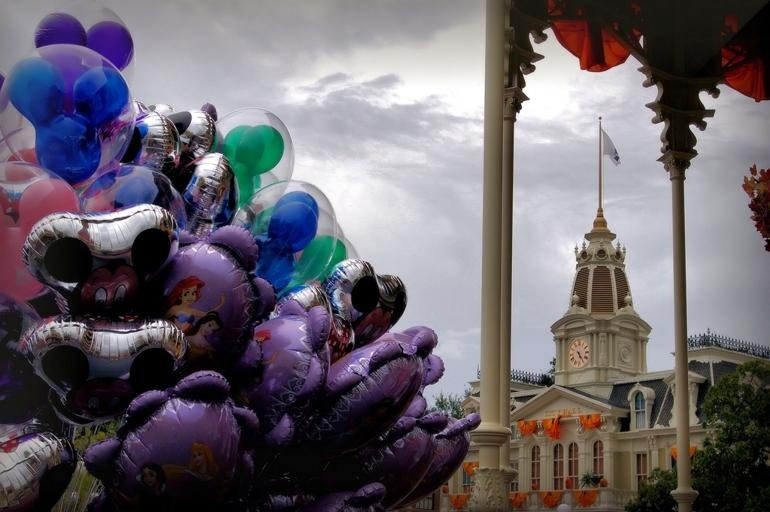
[0,1,482,511]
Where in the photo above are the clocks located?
[567,337,592,369]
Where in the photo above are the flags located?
[601,130,622,167]
